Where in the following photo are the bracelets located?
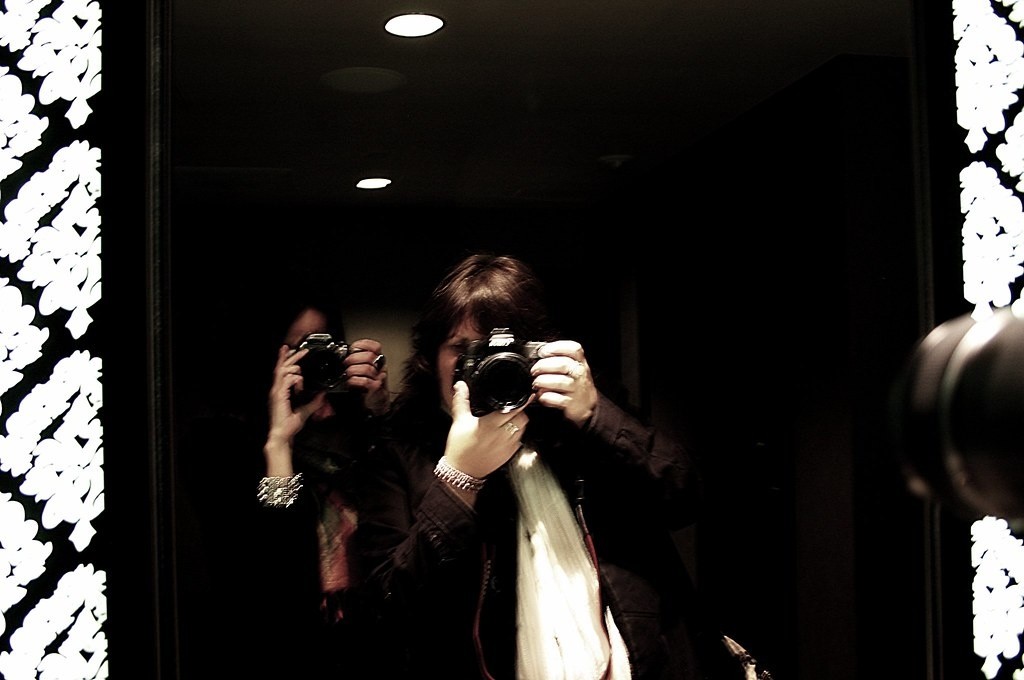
[433,456,487,494]
[256,471,305,507]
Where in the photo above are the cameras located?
[900,309,1024,516]
[454,327,550,416]
[289,332,365,390]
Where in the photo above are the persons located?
[350,251,707,680]
[225,290,409,680]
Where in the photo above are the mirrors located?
[145,0,952,680]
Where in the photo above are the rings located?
[372,353,385,371]
[502,420,519,434]
